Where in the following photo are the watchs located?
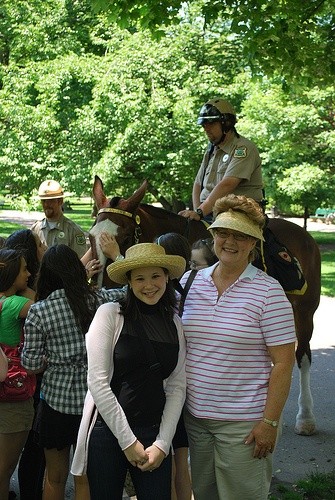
[263,418,279,426]
[195,209,204,219]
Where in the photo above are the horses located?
[88,174,322,436]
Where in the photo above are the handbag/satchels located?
[0,343,36,402]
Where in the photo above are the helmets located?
[39,180,64,199]
[197,99,236,125]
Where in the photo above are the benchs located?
[311,208,334,223]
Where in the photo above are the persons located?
[174,194,296,500]
[0,229,219,500]
[30,180,87,260]
[178,97,265,219]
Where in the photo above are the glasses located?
[215,228,248,241]
[188,261,208,266]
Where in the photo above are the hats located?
[206,194,265,242]
[106,243,186,284]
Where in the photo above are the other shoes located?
[8,491,17,500]
